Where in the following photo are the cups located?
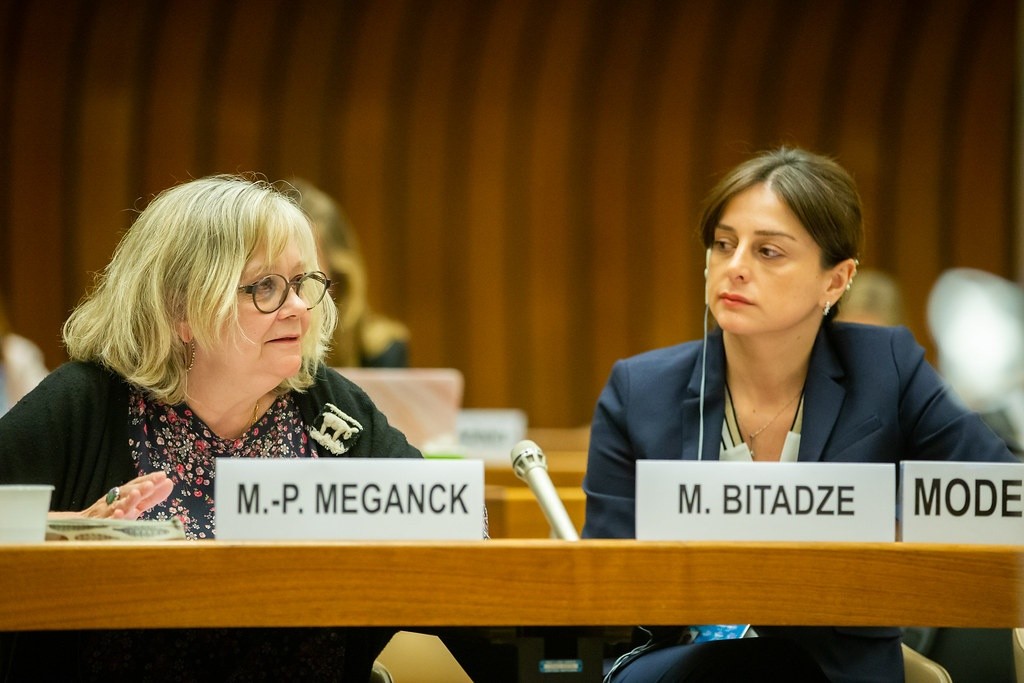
[0,484,54,544]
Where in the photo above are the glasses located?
[238,271,331,314]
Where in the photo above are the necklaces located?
[742,390,804,462]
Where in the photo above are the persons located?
[0,175,539,682]
[274,179,416,371]
[566,149,1024,682]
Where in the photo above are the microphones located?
[511,439,581,543]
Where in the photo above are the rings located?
[106,486,121,506]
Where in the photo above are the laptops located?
[334,366,463,455]
